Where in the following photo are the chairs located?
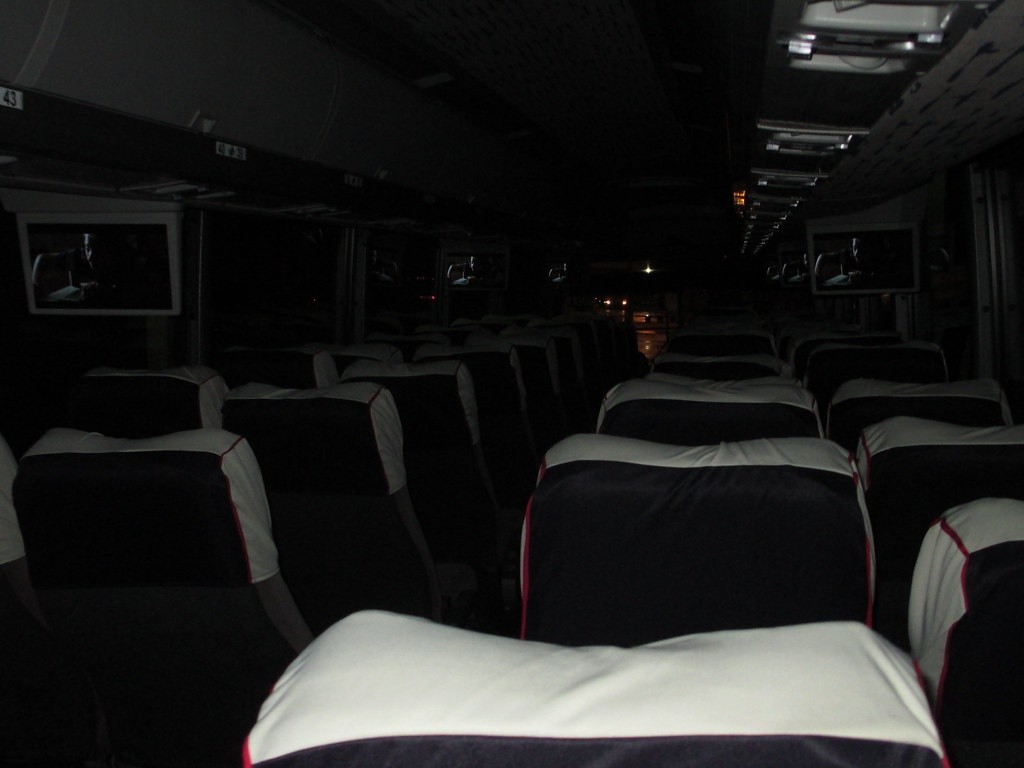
[0,308,1023,768]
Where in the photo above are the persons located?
[817,237,867,289]
[32,232,141,311]
[449,265,464,283]
